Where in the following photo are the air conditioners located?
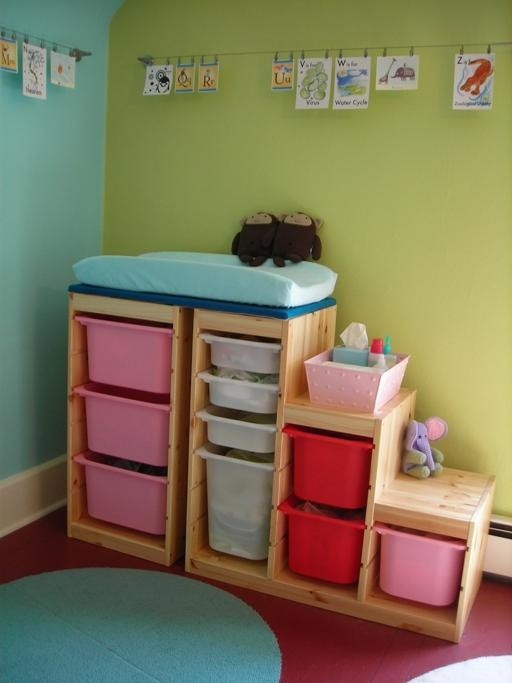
[483,514,512,581]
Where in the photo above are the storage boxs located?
[369,520,469,606]
[276,495,366,585]
[303,349,413,414]
[198,367,279,414]
[75,315,171,395]
[192,443,274,561]
[281,422,375,509]
[72,449,167,537]
[195,405,276,455]
[198,329,282,374]
[72,383,168,467]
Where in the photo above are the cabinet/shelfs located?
[67,284,498,644]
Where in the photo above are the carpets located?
[405,655,512,683]
[0,565,282,681]
